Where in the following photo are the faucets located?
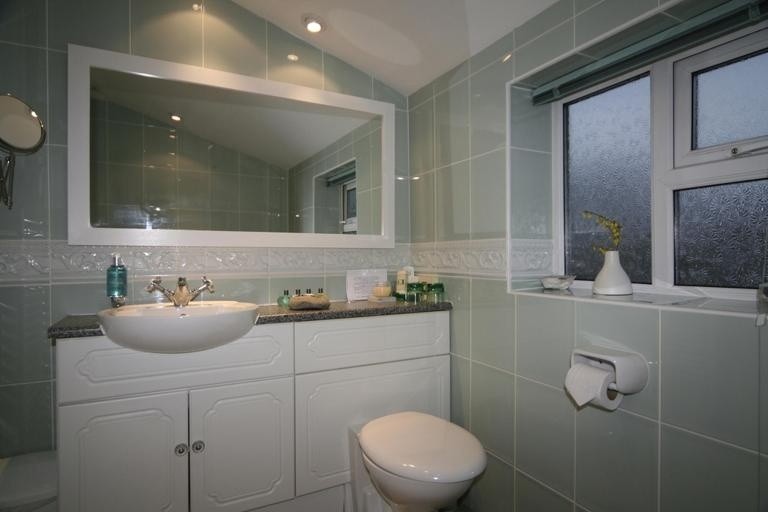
[173,277,191,308]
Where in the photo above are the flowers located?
[578,207,624,256]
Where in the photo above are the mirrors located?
[63,37,395,252]
[1,91,46,211]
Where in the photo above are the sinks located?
[97,301,259,353]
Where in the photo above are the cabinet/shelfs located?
[48,320,293,512]
[294,307,453,512]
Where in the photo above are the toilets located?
[350,412,488,512]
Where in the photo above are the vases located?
[591,248,634,297]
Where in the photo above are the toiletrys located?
[370,271,445,305]
[277,288,324,308]
